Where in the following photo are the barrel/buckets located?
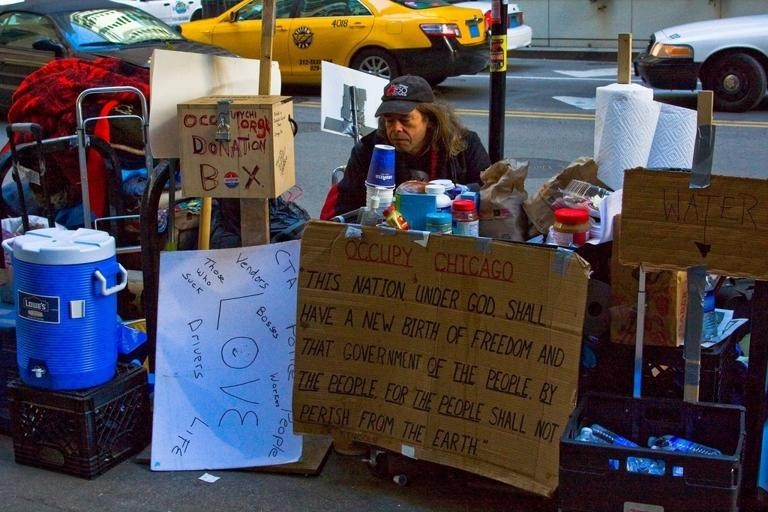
[0,226,129,391]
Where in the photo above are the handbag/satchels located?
[218,198,309,238]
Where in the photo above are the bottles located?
[422,176,481,237]
[575,422,723,478]
[701,273,718,340]
[552,208,590,248]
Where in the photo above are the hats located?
[375,75,434,118]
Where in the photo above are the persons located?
[337,75,490,217]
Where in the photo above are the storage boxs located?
[606,331,737,404]
[7,362,152,480]
[0,340,16,436]
[559,391,747,512]
[176,94,295,199]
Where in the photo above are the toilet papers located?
[593,85,698,191]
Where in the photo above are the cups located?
[363,143,397,209]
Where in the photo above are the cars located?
[178,1,490,84]
[455,0,532,50]
[633,14,767,114]
[0,0,244,122]
[112,0,203,29]
